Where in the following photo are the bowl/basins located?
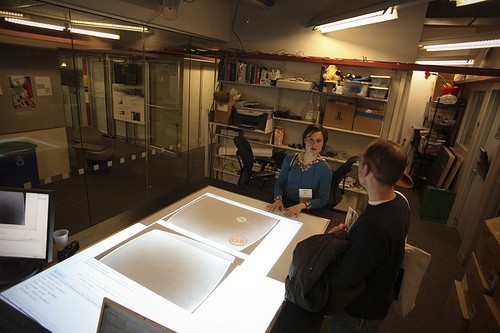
[441,86,459,96]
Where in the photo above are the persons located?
[267,124,331,218]
[324,137,412,333]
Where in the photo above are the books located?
[217,60,281,86]
[423,144,463,195]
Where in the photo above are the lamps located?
[415,30,500,64]
[0,0,150,40]
[307,0,401,34]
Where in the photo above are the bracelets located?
[304,202,311,209]
[273,195,282,201]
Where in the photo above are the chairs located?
[73,125,115,172]
[232,132,278,186]
[327,154,360,208]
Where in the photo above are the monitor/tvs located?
[113,63,139,85]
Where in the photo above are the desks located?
[0,184,332,333]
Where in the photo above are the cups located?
[54,229,69,251]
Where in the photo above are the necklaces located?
[298,151,323,172]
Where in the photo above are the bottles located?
[304,100,315,121]
[312,103,321,123]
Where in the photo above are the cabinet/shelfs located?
[408,96,466,191]
[205,74,391,212]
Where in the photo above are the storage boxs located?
[419,177,456,225]
[274,127,285,144]
[323,99,357,131]
[444,213,500,333]
[368,85,389,99]
[231,103,272,133]
[343,80,369,97]
[353,106,387,138]
[275,79,314,91]
[370,74,391,87]
